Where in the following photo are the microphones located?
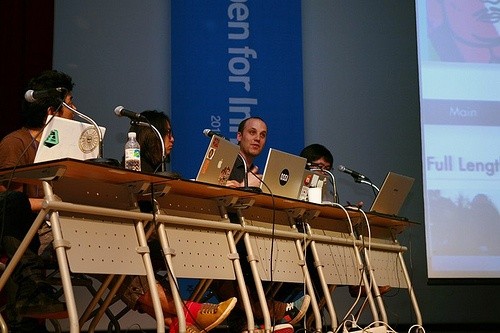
[203,129,219,137]
[306,162,320,166]
[338,165,370,181]
[24,88,66,102]
[114,105,146,120]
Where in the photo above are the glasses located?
[168,130,174,138]
[317,165,334,173]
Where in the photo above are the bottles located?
[124,132,141,172]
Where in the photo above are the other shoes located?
[349,285,391,299]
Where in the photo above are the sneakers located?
[278,295,311,326]
[242,319,294,333]
[169,296,237,333]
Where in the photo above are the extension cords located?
[350,326,387,333]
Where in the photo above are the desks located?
[0,158,423,333]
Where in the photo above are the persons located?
[300,144,392,333]
[121,110,311,333]
[226,118,268,188]
[0,69,237,333]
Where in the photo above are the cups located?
[307,187,322,203]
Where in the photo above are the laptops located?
[369,172,415,216]
[196,135,241,186]
[33,115,106,164]
[260,148,308,199]
